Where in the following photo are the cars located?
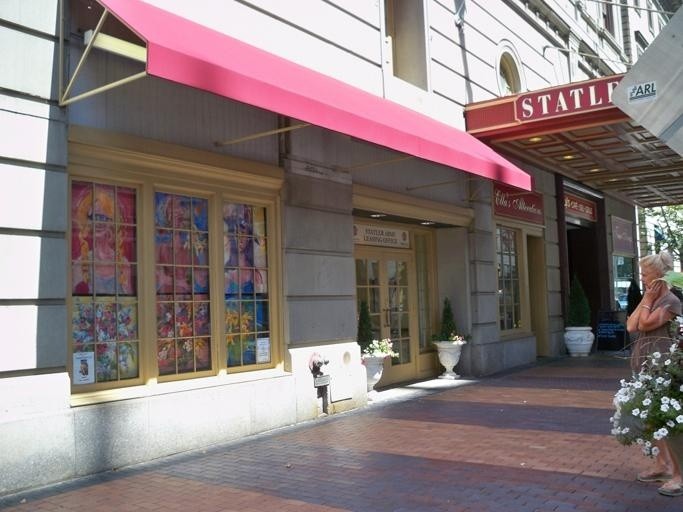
[616,294,628,312]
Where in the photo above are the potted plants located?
[564,273,595,358]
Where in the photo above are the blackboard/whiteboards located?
[596,323,625,351]
[610,215,634,258]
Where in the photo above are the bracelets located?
[642,305,651,310]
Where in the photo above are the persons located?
[626,251,683,497]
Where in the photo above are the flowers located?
[431,295,471,342]
[358,301,400,358]
[599,316,683,454]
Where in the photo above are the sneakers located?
[637,466,674,483]
[657,477,683,497]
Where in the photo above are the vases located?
[434,340,467,379]
[361,355,385,391]
